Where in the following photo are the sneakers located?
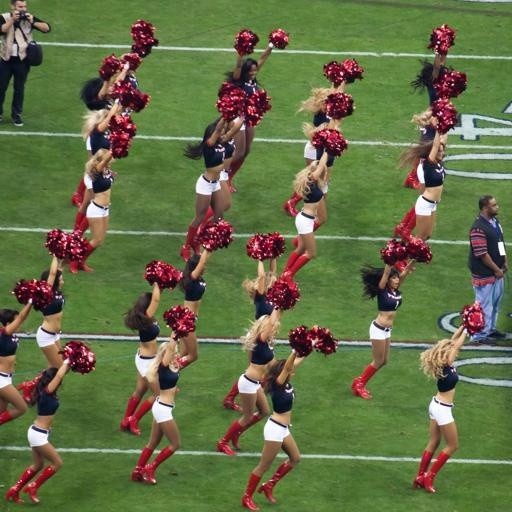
[11,114,24,128]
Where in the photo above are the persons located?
[168,220,232,369]
[17,230,88,403]
[3,341,93,504]
[81,59,130,209]
[352,235,433,398]
[281,89,354,215]
[394,96,459,240]
[314,59,362,181]
[119,261,180,435]
[429,69,468,160]
[179,89,245,261]
[1,0,51,126]
[1,279,52,425]
[275,128,346,291]
[65,130,129,272]
[223,235,287,411]
[221,29,287,192]
[216,274,299,456]
[72,60,137,214]
[242,327,338,508]
[413,303,486,493]
[130,306,197,486]
[466,194,507,343]
[406,25,456,190]
[98,20,159,160]
[385,116,453,271]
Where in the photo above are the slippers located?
[353,364,373,400]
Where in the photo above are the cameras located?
[20,10,26,20]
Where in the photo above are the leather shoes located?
[476,338,496,347]
[489,330,507,340]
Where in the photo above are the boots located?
[1,409,14,427]
[293,206,301,216]
[4,467,37,506]
[290,216,321,246]
[282,191,300,221]
[118,394,142,432]
[191,222,213,258]
[130,446,155,483]
[239,472,262,512]
[179,225,194,264]
[283,249,301,275]
[228,184,234,194]
[79,243,95,273]
[231,413,262,451]
[402,156,423,192]
[400,212,416,245]
[22,464,59,504]
[125,399,144,437]
[392,206,415,245]
[280,254,311,294]
[350,365,370,391]
[411,448,436,489]
[221,382,244,413]
[215,418,239,458]
[72,216,90,238]
[68,240,90,274]
[73,211,85,231]
[16,372,45,406]
[197,206,215,236]
[422,449,451,494]
[232,186,236,193]
[255,460,295,506]
[141,445,175,486]
[70,178,85,208]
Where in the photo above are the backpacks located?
[13,13,44,66]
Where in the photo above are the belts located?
[39,326,60,336]
[201,173,219,184]
[267,414,294,432]
[155,398,176,410]
[241,373,264,387]
[372,320,393,334]
[92,200,111,211]
[433,396,455,409]
[31,425,50,435]
[420,194,443,206]
[0,371,15,379]
[135,349,155,362]
[222,168,231,173]
